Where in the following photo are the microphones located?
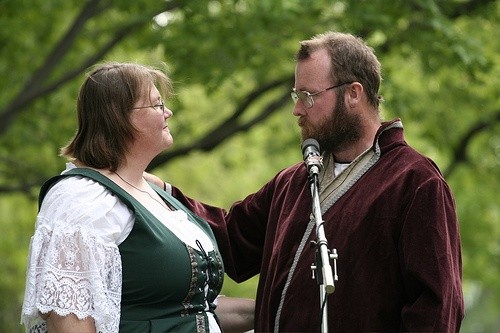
[300,138,323,178]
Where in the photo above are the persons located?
[15,58,227,333]
[120,30,466,333]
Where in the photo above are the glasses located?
[291,82,351,107]
[129,100,165,114]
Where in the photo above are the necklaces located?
[110,164,182,219]
